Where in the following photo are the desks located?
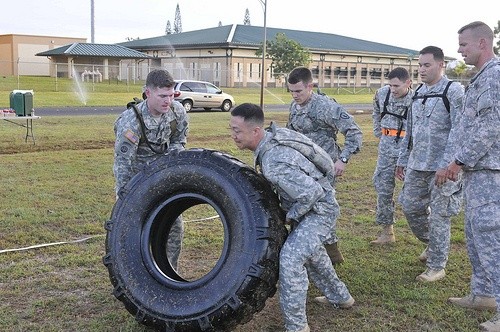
[0,115,42,146]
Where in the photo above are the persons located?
[369,66,420,247]
[283,67,363,268]
[394,45,468,284]
[228,102,356,332]
[110,68,190,274]
[439,20,500,331]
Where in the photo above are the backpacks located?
[259,121,334,188]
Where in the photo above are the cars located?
[174,80,236,112]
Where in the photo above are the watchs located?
[455,159,465,166]
[339,157,349,164]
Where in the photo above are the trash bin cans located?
[10,90,33,115]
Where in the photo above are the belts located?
[381,128,405,138]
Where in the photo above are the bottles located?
[31,108,34,117]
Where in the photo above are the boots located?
[370,224,395,246]
[324,242,345,265]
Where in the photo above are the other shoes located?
[415,267,445,282]
[419,245,430,261]
[315,296,355,308]
[448,294,497,311]
[480,313,500,332]
[285,324,310,332]
